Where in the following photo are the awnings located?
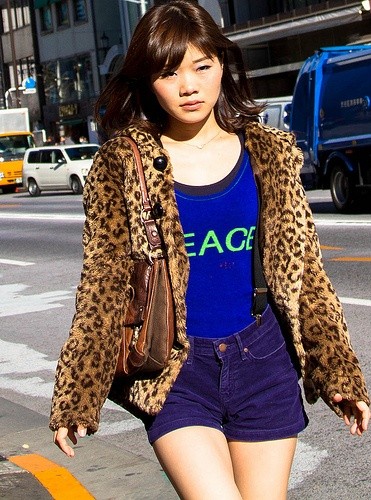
[59,118,83,127]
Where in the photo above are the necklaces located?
[173,131,223,150]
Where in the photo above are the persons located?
[48,0,371,500]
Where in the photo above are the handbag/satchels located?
[101,136,176,379]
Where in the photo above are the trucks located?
[283,43,371,212]
[0,109,39,196]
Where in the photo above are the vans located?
[20,142,103,198]
[230,94,324,195]
